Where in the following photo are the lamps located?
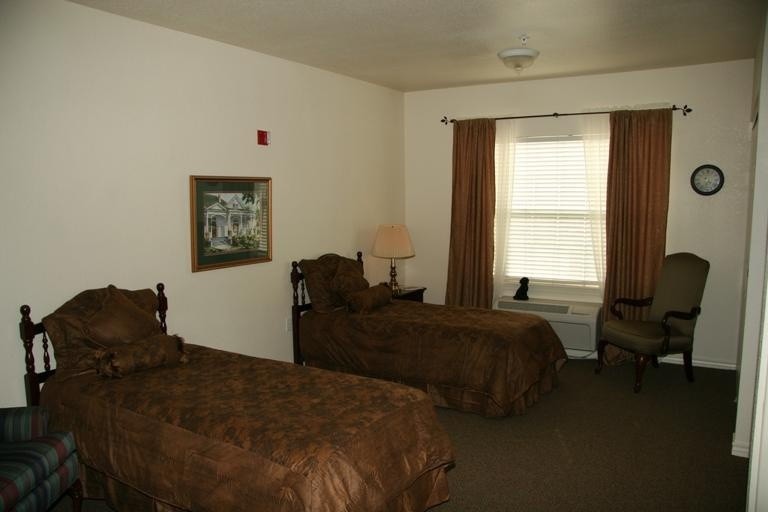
[372,224,417,294]
[499,49,540,71]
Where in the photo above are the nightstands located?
[386,285,428,301]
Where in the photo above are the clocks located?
[689,163,725,196]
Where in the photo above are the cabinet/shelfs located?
[494,295,604,353]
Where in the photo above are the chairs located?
[595,252,710,393]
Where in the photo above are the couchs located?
[0,403,82,512]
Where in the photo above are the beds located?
[290,251,569,420]
[18,282,455,512]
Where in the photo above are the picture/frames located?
[189,174,272,274]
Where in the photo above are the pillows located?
[93,333,191,380]
[332,262,369,301]
[80,284,161,351]
[349,280,393,314]
